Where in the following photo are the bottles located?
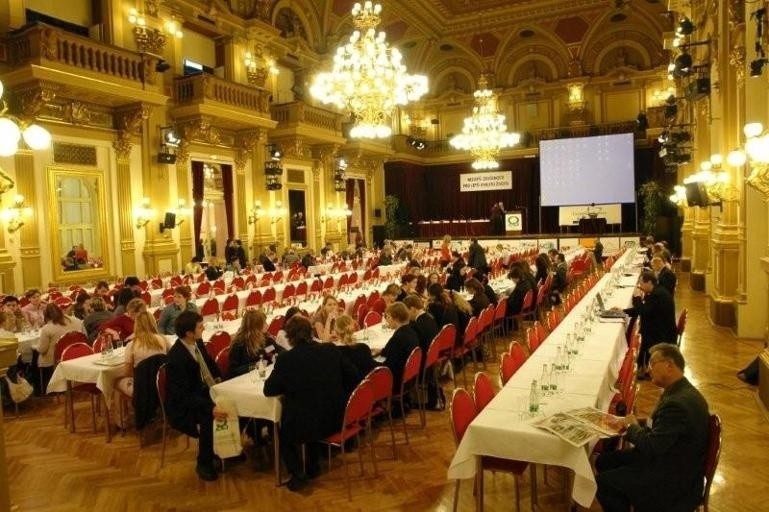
[253,264,514,378]
[526,246,638,420]
[98,253,398,362]
[20,319,42,339]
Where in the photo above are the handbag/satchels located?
[744,357,759,385]
[8,375,34,403]
[408,383,445,411]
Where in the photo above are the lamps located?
[150,127,283,192]
[309,0,430,150]
[136,198,193,231]
[321,200,350,224]
[658,18,769,207]
[332,157,351,190]
[248,203,284,225]
[124,7,281,89]
[0,77,53,240]
[447,0,521,169]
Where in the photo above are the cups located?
[115,341,125,357]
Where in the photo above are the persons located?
[263,316,350,488]
[499,200,507,231]
[596,341,709,512]
[164,313,227,480]
[2,233,675,459]
[488,201,506,235]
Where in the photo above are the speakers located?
[685,181,708,207]
[164,212,175,229]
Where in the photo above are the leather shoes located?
[196,462,216,481]
[638,373,652,380]
[286,463,319,488]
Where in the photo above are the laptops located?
[596,292,626,318]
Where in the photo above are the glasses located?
[649,359,669,369]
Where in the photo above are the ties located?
[195,345,216,387]
